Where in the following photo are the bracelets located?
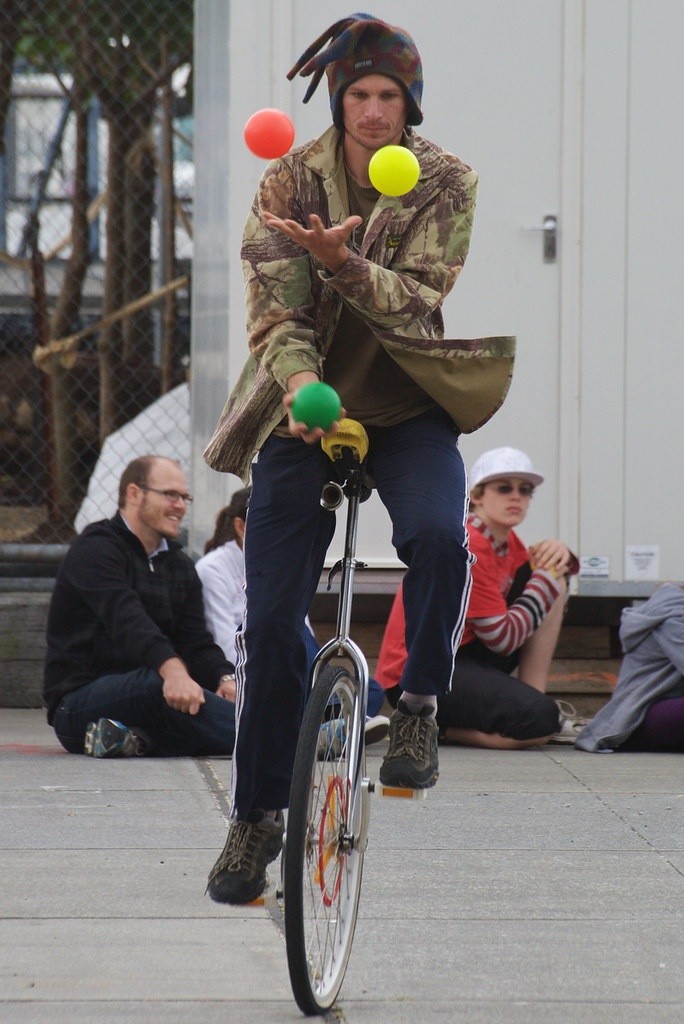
[219,673,235,685]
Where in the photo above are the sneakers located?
[378,700,441,790]
[83,719,142,757]
[203,809,285,903]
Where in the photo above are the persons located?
[195,486,393,750]
[40,458,236,759]
[202,13,516,906]
[373,448,598,747]
[576,580,684,753]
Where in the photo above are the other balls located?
[290,380,343,432]
[368,144,421,197]
[244,109,295,161]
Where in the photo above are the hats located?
[286,13,423,131]
[468,448,544,491]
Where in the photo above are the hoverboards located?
[238,413,430,1018]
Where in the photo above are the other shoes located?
[315,715,349,761]
[363,713,390,746]
[551,717,583,744]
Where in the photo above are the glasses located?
[482,482,532,496]
[137,484,194,506]
